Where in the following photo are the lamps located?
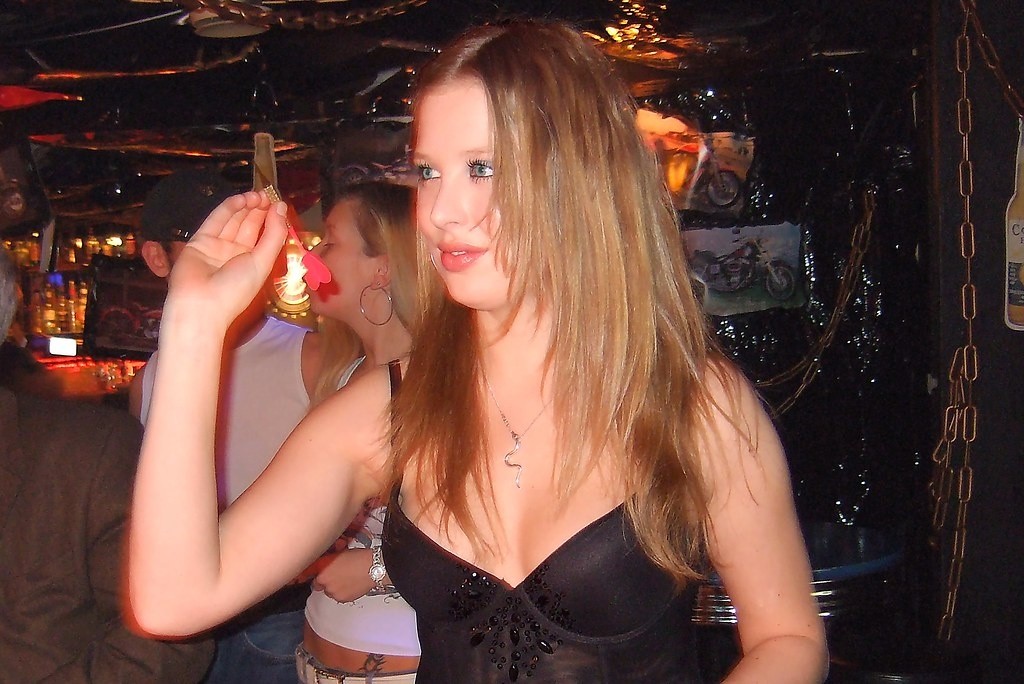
[190,3,273,38]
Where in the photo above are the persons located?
[129,17,828,683]
[0,167,422,684]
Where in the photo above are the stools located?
[691,523,908,684]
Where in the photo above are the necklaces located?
[484,369,553,489]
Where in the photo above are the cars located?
[685,501,956,684]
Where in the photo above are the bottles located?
[32,279,87,334]
[75,360,135,390]
[0,231,137,274]
[1004,115,1024,331]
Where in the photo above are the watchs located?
[368,546,386,588]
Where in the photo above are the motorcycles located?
[682,130,744,211]
[685,230,795,303]
